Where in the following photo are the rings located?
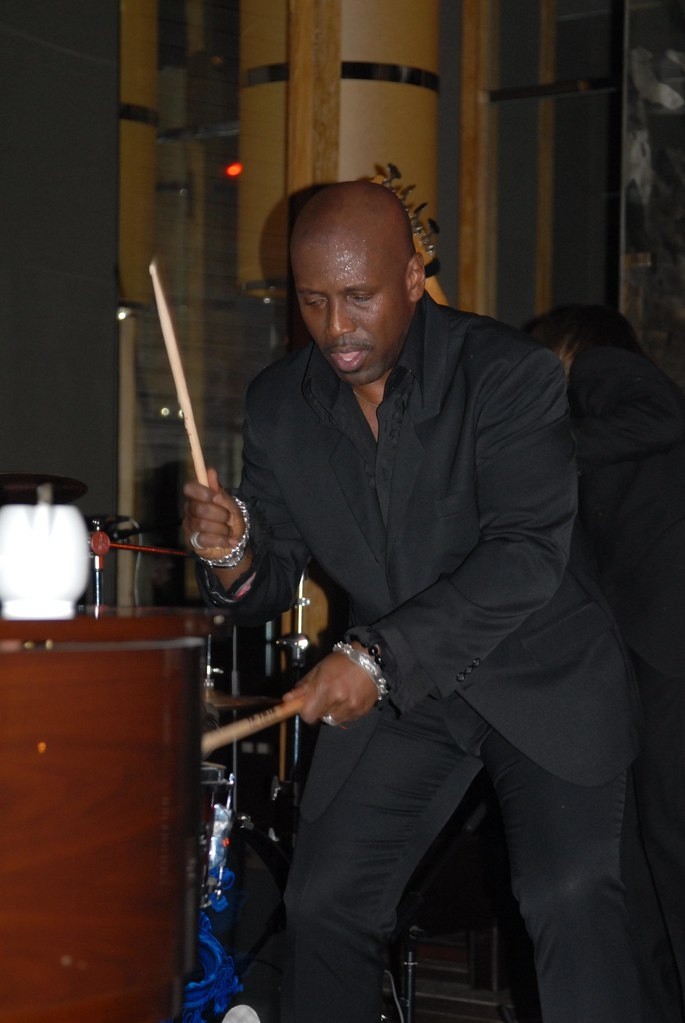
[321,713,339,727]
[190,531,205,551]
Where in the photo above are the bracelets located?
[201,494,250,569]
[331,633,391,707]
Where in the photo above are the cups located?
[0,502,89,620]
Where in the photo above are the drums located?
[198,760,234,910]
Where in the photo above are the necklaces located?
[353,390,378,407]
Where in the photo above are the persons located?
[182,181,685,1023]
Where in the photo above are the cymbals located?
[85,514,147,542]
[0,470,90,509]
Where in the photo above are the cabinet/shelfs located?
[0,604,237,1023]
